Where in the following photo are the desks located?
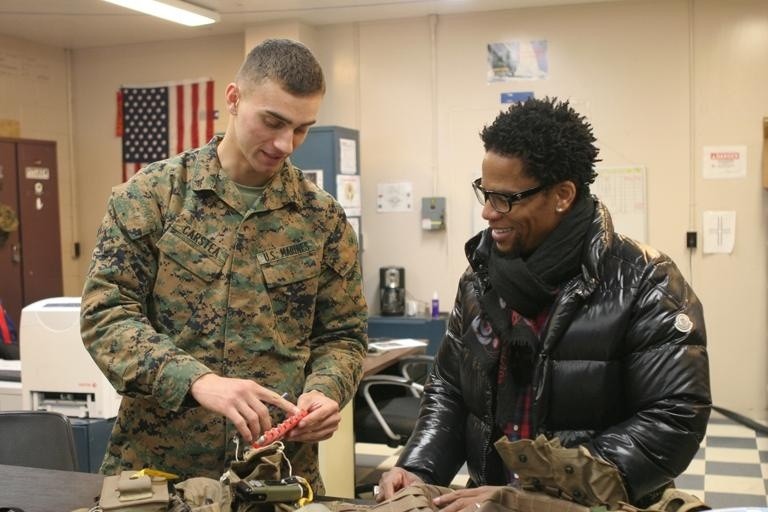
[363,338,432,383]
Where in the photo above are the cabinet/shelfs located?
[366,312,446,345]
[290,126,362,257]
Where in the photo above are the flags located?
[120,78,215,186]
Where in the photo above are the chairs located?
[0,410,80,473]
[359,355,437,442]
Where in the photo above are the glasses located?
[470,177,550,213]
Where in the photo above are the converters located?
[687,231,697,248]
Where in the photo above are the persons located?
[79,35,369,497]
[373,94,712,511]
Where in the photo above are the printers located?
[18,295,124,421]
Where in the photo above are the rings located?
[474,502,482,511]
